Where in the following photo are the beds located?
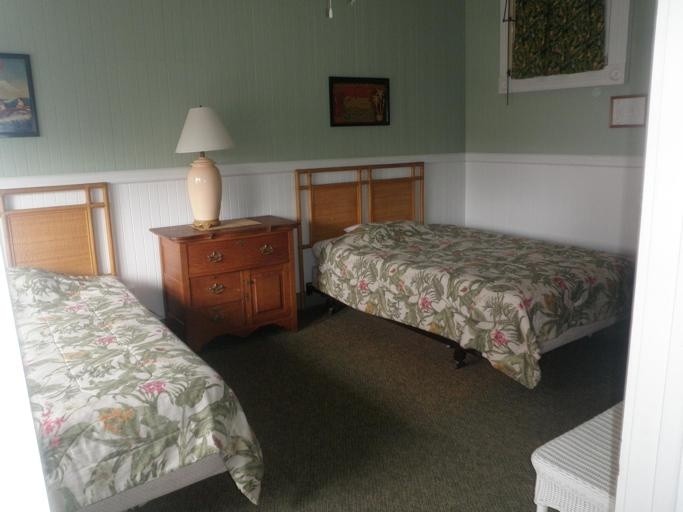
[0,184,237,512]
[291,162,632,367]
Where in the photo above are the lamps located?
[171,105,237,228]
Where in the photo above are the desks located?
[528,388,622,512]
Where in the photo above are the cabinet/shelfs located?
[149,215,305,353]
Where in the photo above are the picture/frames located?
[329,76,392,128]
[0,53,41,139]
[499,2,628,100]
